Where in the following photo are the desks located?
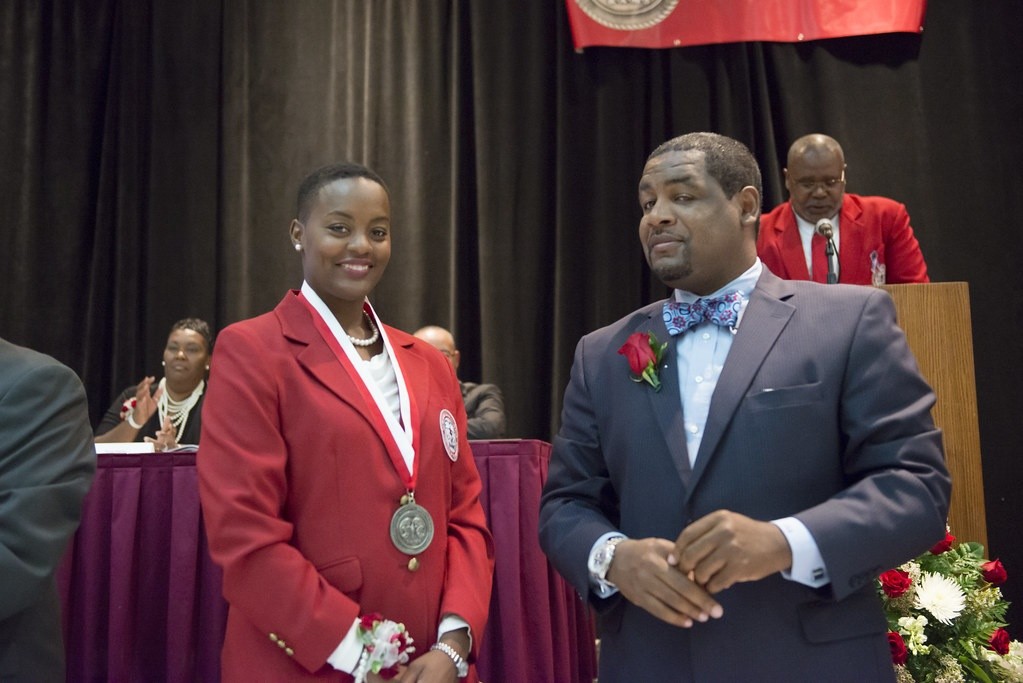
[57,439,597,682]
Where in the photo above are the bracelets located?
[354,613,415,683]
[120,397,143,429]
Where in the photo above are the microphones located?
[816,219,834,240]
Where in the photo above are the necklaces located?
[158,377,204,444]
[347,309,379,346]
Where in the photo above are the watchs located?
[430,643,468,677]
[588,538,627,587]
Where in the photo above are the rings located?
[161,443,168,451]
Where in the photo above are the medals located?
[390,503,434,555]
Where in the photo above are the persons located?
[537,133,953,683]
[0,337,97,683]
[95,318,211,453]
[756,134,930,285]
[199,163,495,683]
[413,326,506,440]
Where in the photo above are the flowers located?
[879,525,1023,683]
[618,328,669,393]
